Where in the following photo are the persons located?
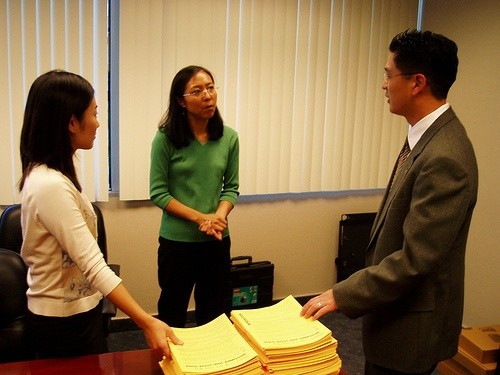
[149,65,239,329]
[18,71,184,361]
[300,29,478,375]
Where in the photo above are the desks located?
[0,346,348,375]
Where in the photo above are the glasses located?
[384,73,417,84]
[183,86,219,97]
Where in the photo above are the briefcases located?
[230,255,274,310]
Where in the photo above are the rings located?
[318,303,322,307]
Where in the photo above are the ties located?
[388,140,411,195]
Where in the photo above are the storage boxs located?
[436,321,500,375]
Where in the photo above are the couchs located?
[0,204,106,360]
